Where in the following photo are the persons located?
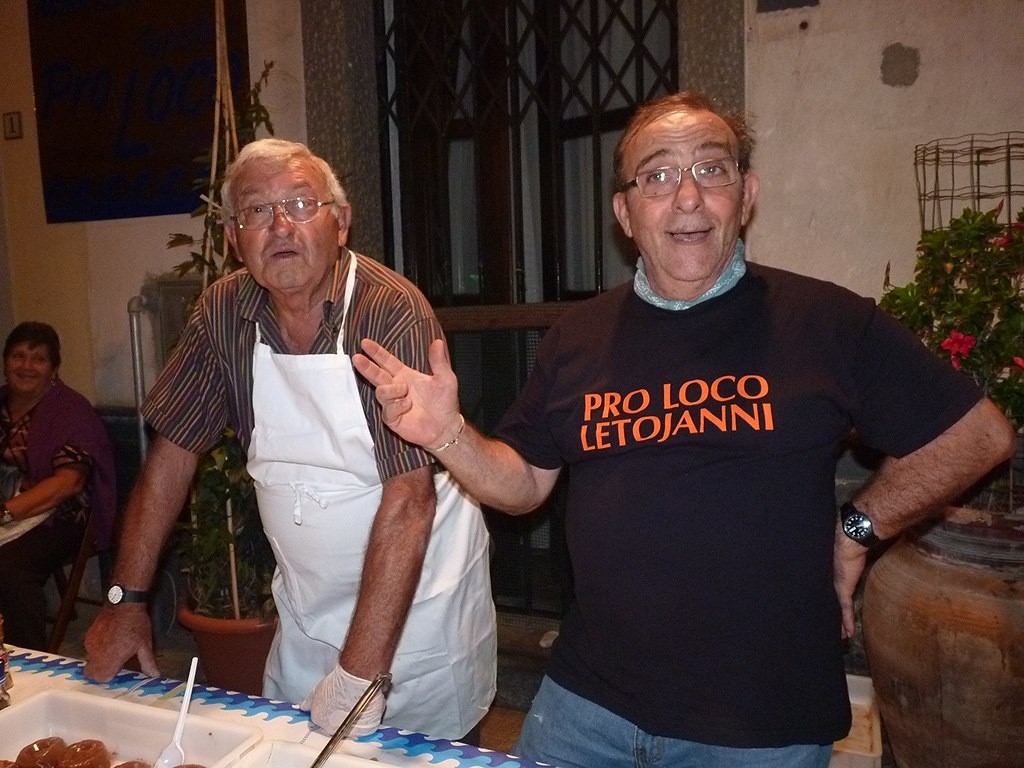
[83,137,495,746]
[351,91,1017,768]
[0,321,119,654]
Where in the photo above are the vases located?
[861,505,1024,768]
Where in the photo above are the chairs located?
[39,508,111,653]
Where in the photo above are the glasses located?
[617,152,746,203]
[227,198,341,230]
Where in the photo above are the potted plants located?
[166,60,279,695]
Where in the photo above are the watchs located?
[105,582,148,605]
[0,504,13,523]
[841,501,881,550]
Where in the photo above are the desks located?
[0,490,58,548]
[0,641,558,768]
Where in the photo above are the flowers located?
[876,198,1024,513]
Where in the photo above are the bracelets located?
[423,414,464,452]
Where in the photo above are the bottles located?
[0,614,13,710]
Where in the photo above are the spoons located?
[152,656,199,768]
